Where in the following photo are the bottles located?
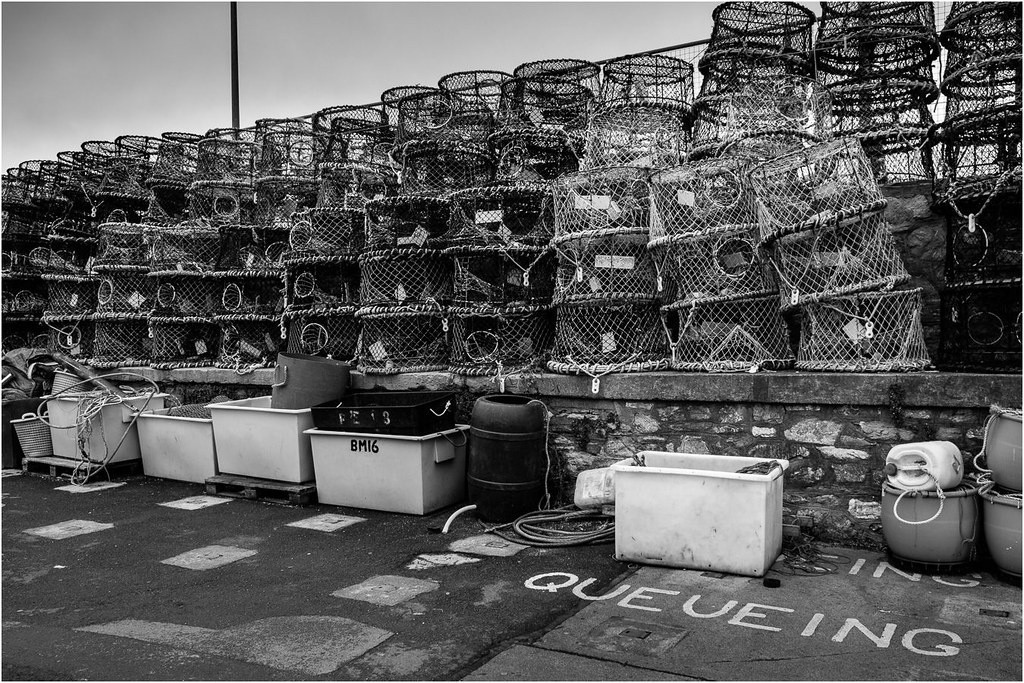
[882,440,963,492]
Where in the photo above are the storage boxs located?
[609,450,790,578]
[2,390,472,516]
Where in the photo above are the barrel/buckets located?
[464,394,548,522]
[270,351,353,410]
